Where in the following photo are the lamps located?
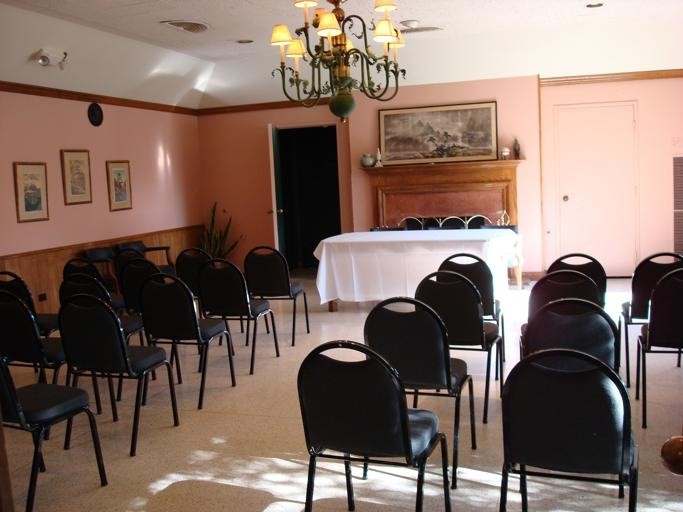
[269,1,407,124]
[32,46,67,73]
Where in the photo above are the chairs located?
[520,297,621,376]
[546,253,608,300]
[1,243,310,457]
[522,270,603,340]
[297,337,453,511]
[620,251,683,389]
[415,270,503,424]
[2,359,109,511]
[634,269,683,429]
[499,347,639,512]
[362,296,478,490]
[437,253,505,381]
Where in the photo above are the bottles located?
[360,154,375,166]
[500,147,510,159]
[513,139,519,159]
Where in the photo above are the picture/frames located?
[106,160,132,212]
[12,161,50,223]
[59,150,92,206]
[378,101,498,166]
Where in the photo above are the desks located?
[320,228,513,313]
